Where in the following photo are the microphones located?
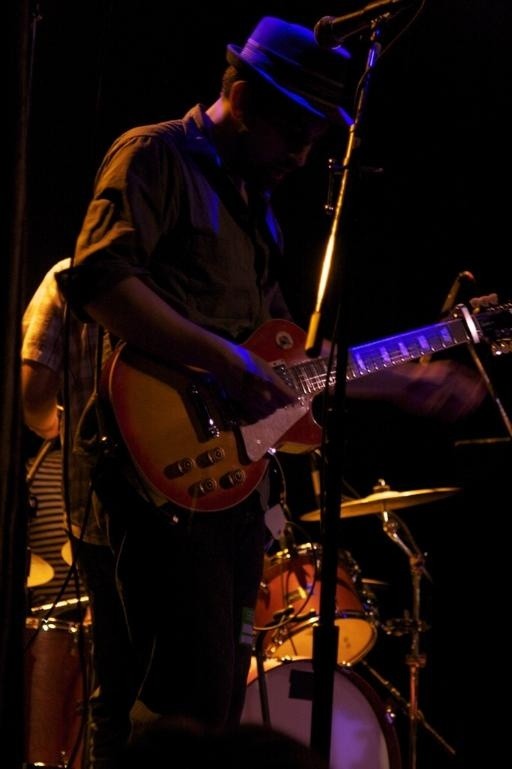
[314,0,406,49]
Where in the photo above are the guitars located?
[99,295,512,513]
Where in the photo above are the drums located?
[24,618,95,768]
[241,657,402,768]
[253,543,377,667]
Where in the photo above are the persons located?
[65,11,492,765]
[18,248,122,768]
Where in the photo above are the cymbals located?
[300,487,459,521]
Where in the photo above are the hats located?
[225,16,358,130]
